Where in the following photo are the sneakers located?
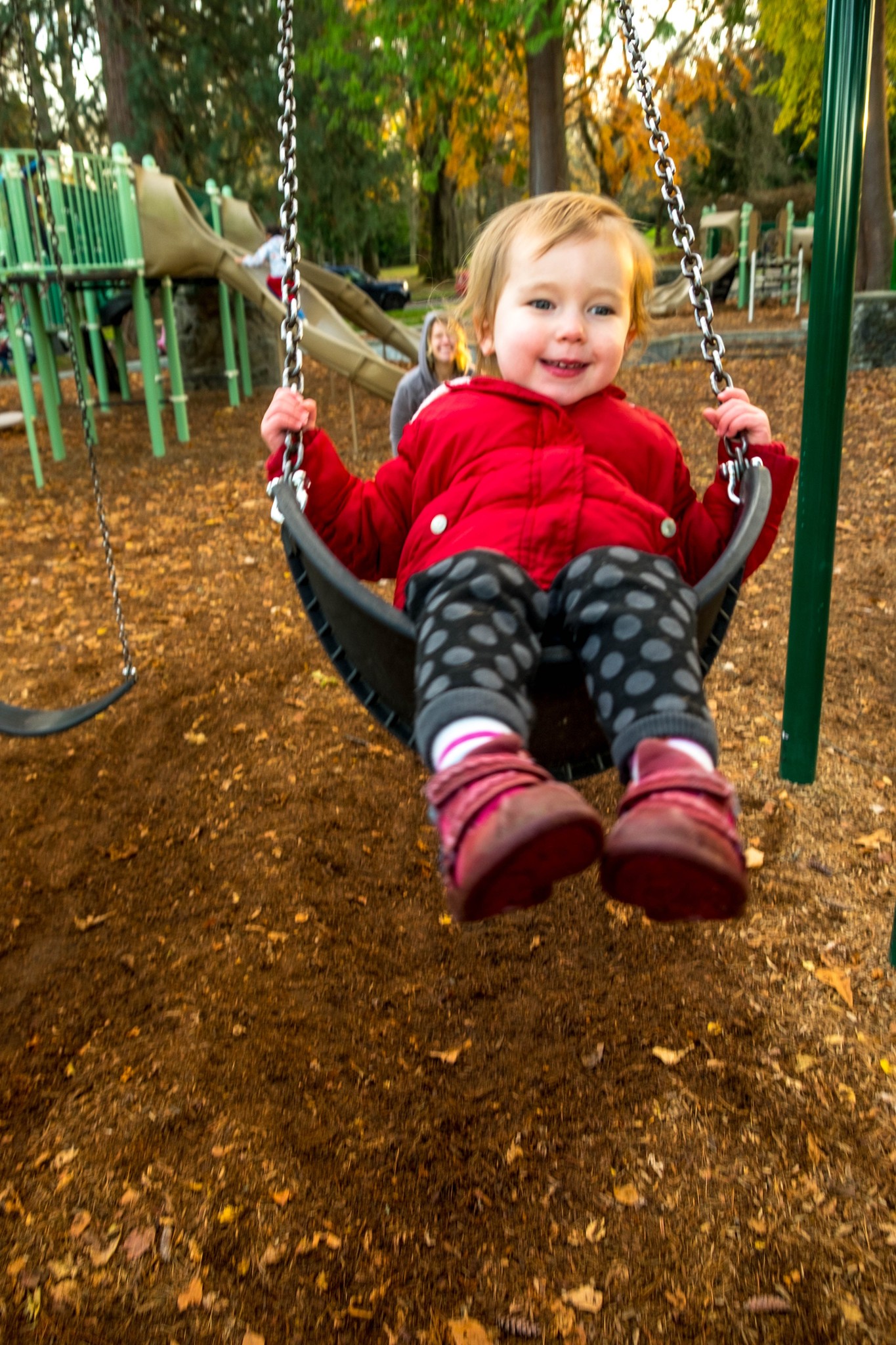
[599,740,751,925]
[427,736,600,922]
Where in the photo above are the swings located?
[0,1,142,738]
[266,1,772,781]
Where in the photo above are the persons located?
[260,191,801,925]
[0,339,14,379]
[22,328,36,372]
[236,222,308,325]
[389,309,476,459]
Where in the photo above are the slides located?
[221,198,421,365]
[644,252,740,317]
[137,168,409,405]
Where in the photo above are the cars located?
[322,264,411,312]
